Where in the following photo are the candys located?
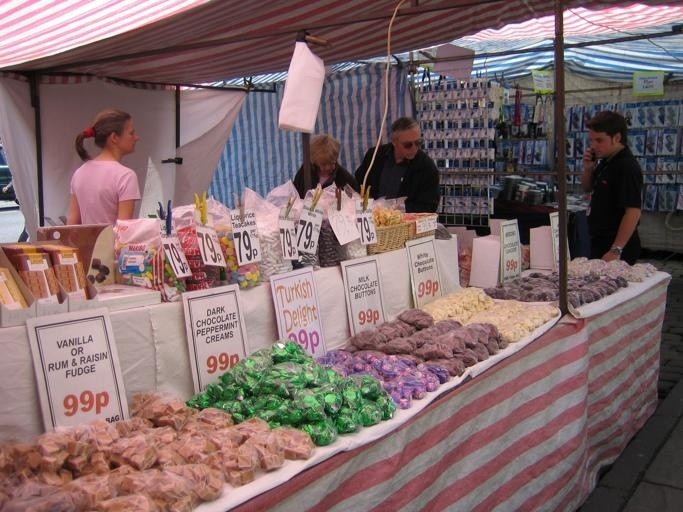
[215,224,260,290]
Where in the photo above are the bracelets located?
[610,244,623,254]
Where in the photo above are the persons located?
[582,111,644,263]
[293,135,356,199]
[356,117,440,214]
[65,109,142,225]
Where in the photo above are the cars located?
[0,145,20,207]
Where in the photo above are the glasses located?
[396,138,424,149]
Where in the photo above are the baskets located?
[403,212,438,240]
[366,222,405,255]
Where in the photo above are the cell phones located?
[590,152,597,162]
